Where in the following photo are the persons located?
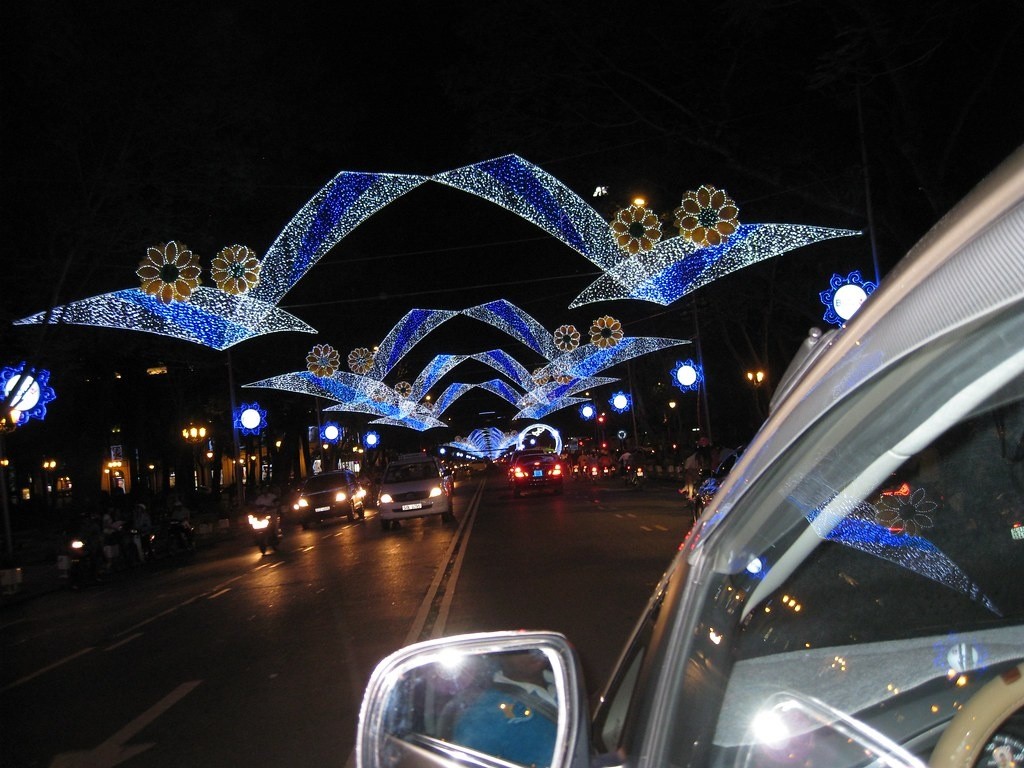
[454,649,557,768]
[102,501,122,573]
[255,485,280,546]
[134,503,152,526]
[172,502,190,523]
[561,437,737,487]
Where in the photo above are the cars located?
[511,453,566,498]
[377,453,456,530]
[503,449,546,490]
[292,468,366,529]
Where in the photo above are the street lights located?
[181,419,212,490]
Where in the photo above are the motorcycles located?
[246,504,284,555]
[572,466,646,491]
[66,514,194,588]
